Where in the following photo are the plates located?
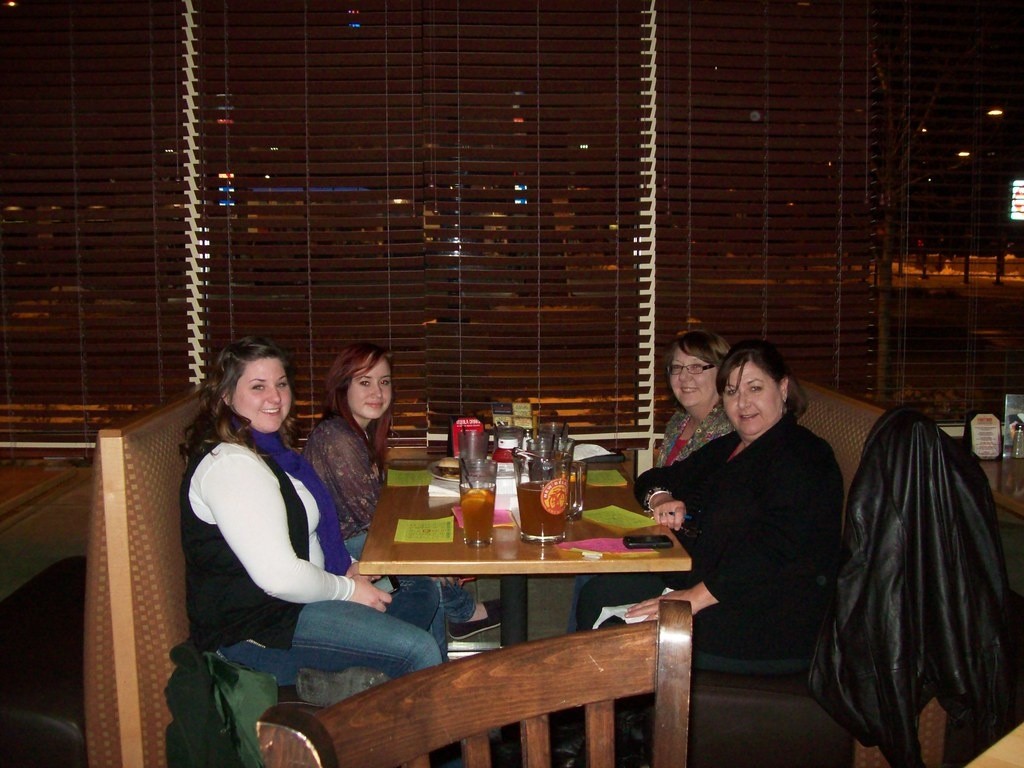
[428,458,462,482]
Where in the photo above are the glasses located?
[667,363,717,376]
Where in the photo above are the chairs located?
[255,600,690,768]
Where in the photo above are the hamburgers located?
[435,457,461,479]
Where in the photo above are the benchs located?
[660,385,946,768]
[84,400,217,768]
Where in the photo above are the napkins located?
[573,444,616,460]
[427,479,460,497]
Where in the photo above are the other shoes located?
[447,598,501,640]
[544,710,652,768]
[296,665,390,706]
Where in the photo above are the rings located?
[659,512,667,516]
[655,613,659,618]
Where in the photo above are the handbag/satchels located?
[201,648,279,768]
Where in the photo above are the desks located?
[977,457,1024,517]
[0,461,97,596]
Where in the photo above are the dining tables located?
[358,452,692,768]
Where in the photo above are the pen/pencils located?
[669,511,693,521]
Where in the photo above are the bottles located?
[1011,422,1024,458]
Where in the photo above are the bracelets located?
[649,491,670,512]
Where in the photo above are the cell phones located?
[624,535,674,549]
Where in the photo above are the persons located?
[181,339,503,768]
[554,331,845,768]
[306,342,502,663]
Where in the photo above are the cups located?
[526,422,575,464]
[552,461,586,522]
[457,457,497,549]
[511,449,571,543]
[457,431,489,469]
